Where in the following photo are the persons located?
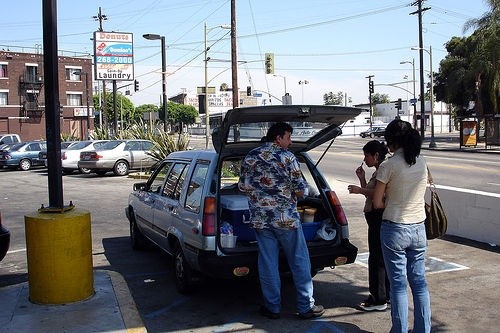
[372,118,440,333]
[237,120,326,320]
[347,139,392,312]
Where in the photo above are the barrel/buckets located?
[302,222,320,241]
[220,231,238,248]
[297,206,318,223]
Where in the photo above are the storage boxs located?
[222,195,256,242]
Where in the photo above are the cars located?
[360,127,385,138]
[0,140,77,171]
[79,139,161,176]
[45,140,110,174]
[129,104,362,292]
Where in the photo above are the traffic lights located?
[127,112,129,120]
[247,87,251,96]
[135,79,139,92]
[395,98,402,110]
[369,81,374,93]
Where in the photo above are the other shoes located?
[259,304,281,319]
[298,304,325,318]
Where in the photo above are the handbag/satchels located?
[425,191,448,240]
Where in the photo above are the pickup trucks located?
[0,133,21,143]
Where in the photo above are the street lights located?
[411,48,435,144]
[400,61,417,130]
[274,75,286,94]
[143,34,166,136]
[299,80,308,105]
[403,75,409,121]
[204,22,231,150]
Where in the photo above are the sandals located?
[356,294,392,311]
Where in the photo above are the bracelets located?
[360,188,361,194]
[362,187,364,194]
